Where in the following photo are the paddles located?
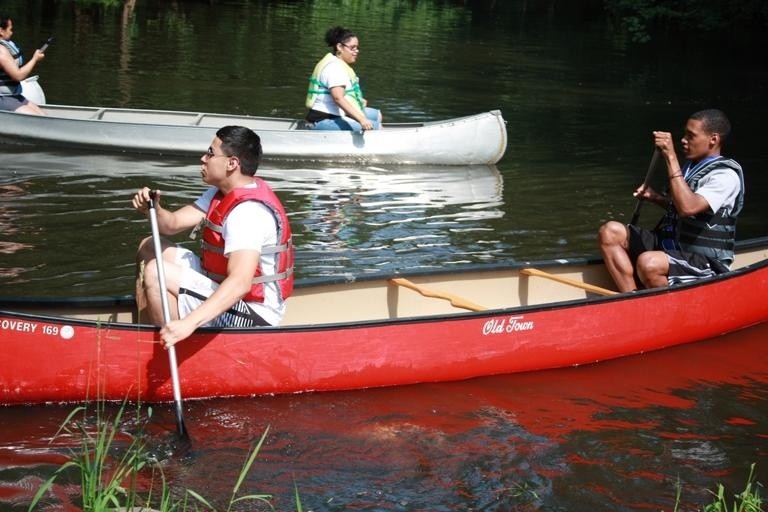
[144,188,194,441]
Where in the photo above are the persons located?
[131,125,296,351]
[306,163,377,264]
[0,14,50,117]
[0,135,30,279]
[596,107,746,296]
[303,25,384,131]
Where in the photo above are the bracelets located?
[667,168,683,179]
[667,173,684,180]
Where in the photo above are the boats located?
[0,100,506,167]
[0,233,768,407]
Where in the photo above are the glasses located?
[340,41,359,50]
[207,147,232,159]
